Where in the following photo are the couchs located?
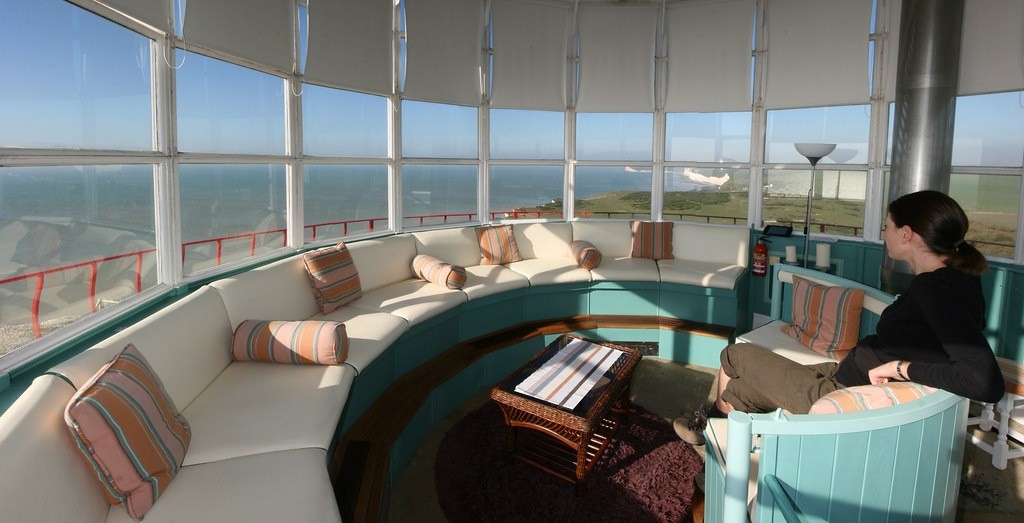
[0,216,1024,523]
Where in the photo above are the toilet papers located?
[785,246,797,262]
[815,243,831,267]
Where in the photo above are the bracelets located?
[897,360,910,382]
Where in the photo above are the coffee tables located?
[488,332,644,498]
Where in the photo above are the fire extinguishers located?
[751,234,772,277]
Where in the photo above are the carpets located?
[433,398,703,523]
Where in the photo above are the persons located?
[673,191,1005,495]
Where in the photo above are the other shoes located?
[672,402,707,445]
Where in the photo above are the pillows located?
[808,376,940,416]
[780,274,869,360]
[229,319,350,367]
[62,342,192,521]
[566,238,603,268]
[409,253,467,291]
[300,240,363,315]
[630,220,675,261]
[474,224,523,266]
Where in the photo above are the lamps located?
[792,142,838,267]
